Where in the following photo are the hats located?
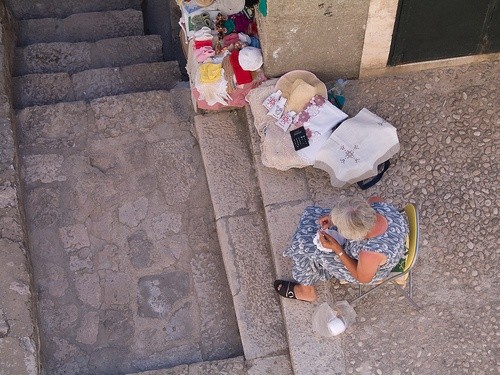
[217,0,244,15]
[275,69,328,115]
[238,46,264,71]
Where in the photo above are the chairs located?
[349,203,421,312]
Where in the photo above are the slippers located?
[274,280,312,303]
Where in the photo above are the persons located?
[274,197,407,304]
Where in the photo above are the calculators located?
[290,126,310,151]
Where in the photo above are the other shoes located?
[191,11,216,63]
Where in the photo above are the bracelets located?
[338,250,345,257]
